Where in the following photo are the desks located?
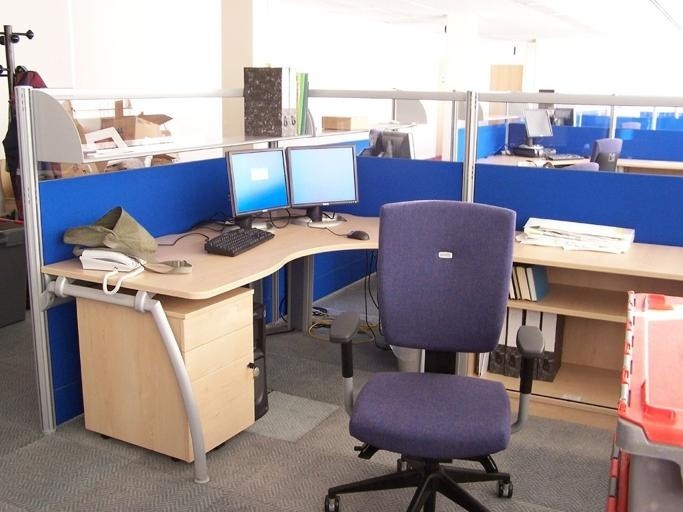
[40,209,380,482]
[477,154,591,168]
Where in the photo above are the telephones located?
[79,247,142,272]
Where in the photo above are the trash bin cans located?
[0,218,26,329]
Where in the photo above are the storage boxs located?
[101,114,172,140]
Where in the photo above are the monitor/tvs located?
[379,130,416,160]
[554,107,575,126]
[518,108,553,149]
[590,137,624,172]
[285,144,360,228]
[224,147,291,228]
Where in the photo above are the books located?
[507,263,550,304]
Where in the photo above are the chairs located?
[324,200,545,511]
[559,138,623,172]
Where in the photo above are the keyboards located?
[548,152,585,161]
[204,227,276,257]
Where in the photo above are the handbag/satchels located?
[63,205,157,257]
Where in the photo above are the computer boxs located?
[252,301,269,422]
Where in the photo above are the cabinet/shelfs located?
[467,226,683,432]
[75,287,256,464]
[615,158,683,176]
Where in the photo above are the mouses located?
[346,230,369,240]
